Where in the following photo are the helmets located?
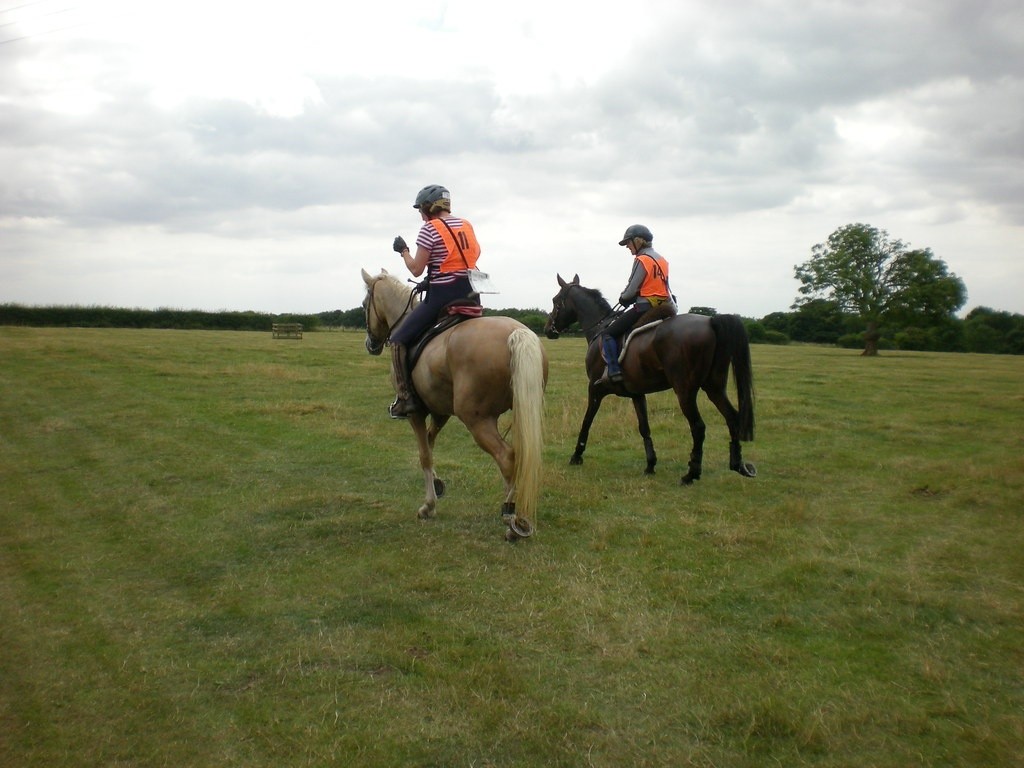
[619,224,653,246]
[413,185,451,217]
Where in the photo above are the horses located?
[360,267,548,541]
[543,273,757,486]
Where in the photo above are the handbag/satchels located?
[467,269,500,295]
[671,293,679,311]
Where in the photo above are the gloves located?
[393,236,409,257]
[619,298,629,307]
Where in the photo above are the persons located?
[594,225,678,388]
[387,185,483,420]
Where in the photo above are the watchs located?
[401,248,408,257]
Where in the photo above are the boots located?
[388,341,415,418]
[593,334,623,386]
